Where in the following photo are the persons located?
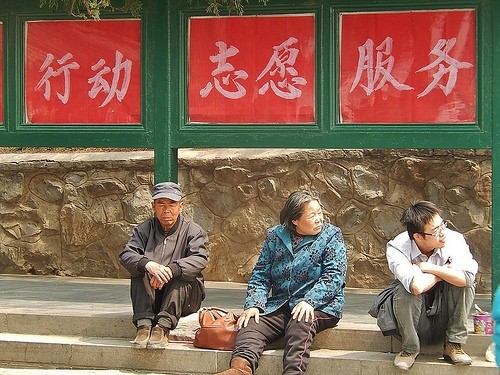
[215,191,347,375]
[119,182,209,350]
[385,201,479,370]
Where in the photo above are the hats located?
[151,182,182,201]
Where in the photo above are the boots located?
[214,357,252,375]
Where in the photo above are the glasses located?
[414,221,447,238]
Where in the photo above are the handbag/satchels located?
[193,308,239,350]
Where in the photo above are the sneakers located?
[393,350,419,370]
[443,342,472,365]
[147,327,169,350]
[132,325,152,349]
